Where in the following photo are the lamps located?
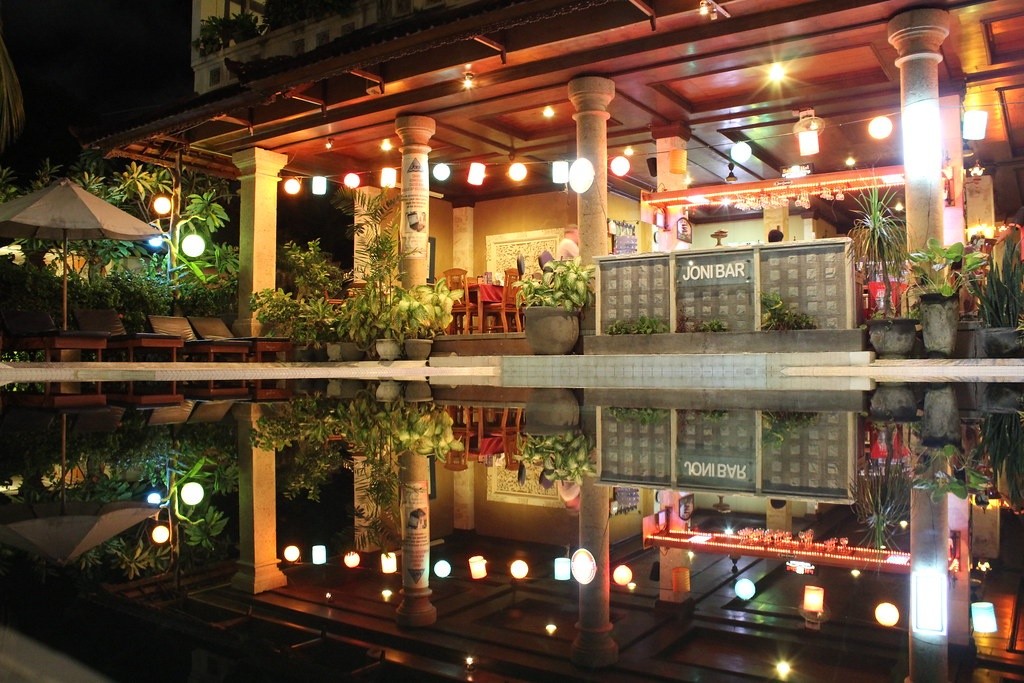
[963,138,973,156]
[726,163,737,182]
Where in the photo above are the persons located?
[557,479,580,511]
[768,230,785,243]
[556,224,579,259]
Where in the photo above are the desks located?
[462,285,504,334]
[470,436,517,456]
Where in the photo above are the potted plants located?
[844,157,1024,358]
[218,188,464,361]
[250,380,465,556]
[848,382,1024,582]
[525,389,598,485]
[513,256,597,354]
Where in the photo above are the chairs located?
[0,379,297,434]
[442,406,522,471]
[0,309,291,362]
[444,268,525,335]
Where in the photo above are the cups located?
[477,276,483,284]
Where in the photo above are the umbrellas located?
[0,178,164,331]
[0,414,161,560]
